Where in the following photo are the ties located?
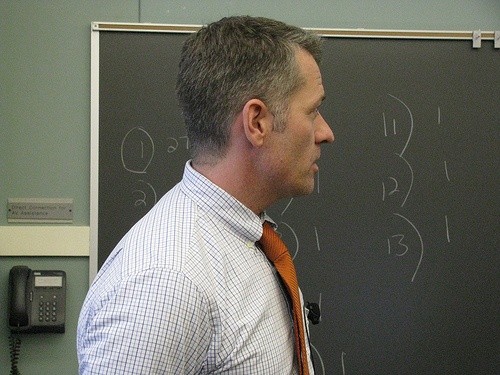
[257,221,309,375]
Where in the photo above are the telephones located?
[8,265,68,336]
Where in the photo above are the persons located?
[76,16,336,375]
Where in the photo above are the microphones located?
[307,302,320,325]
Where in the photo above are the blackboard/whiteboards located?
[87,20,499,374]
